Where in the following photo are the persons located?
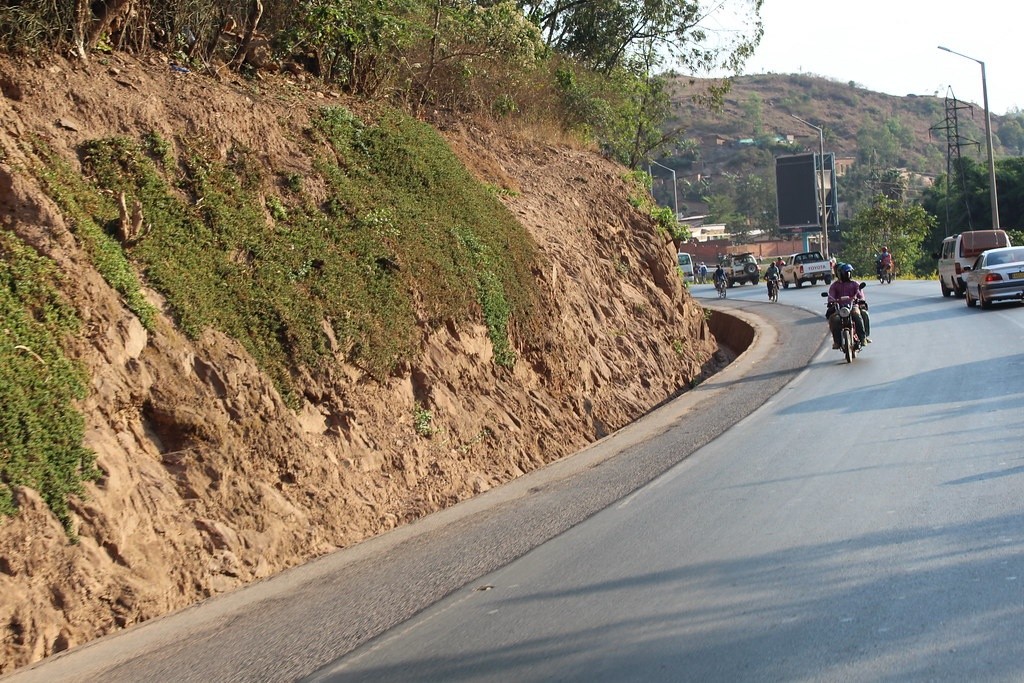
[825,262,872,350]
[877,246,889,274]
[776,256,785,268]
[693,263,699,284]
[764,261,780,300]
[716,265,726,282]
[700,262,707,284]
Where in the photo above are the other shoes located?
[860,338,867,346]
[877,275,880,278]
[866,337,872,343]
[832,342,841,349]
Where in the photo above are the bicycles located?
[767,279,778,302]
[718,279,726,299]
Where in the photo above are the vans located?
[931,229,1015,299]
[678,253,694,282]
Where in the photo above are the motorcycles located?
[821,282,866,364]
[874,254,892,284]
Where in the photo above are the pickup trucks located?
[779,251,832,289]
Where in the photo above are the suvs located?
[716,254,759,289]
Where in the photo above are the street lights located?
[649,158,681,254]
[790,114,830,261]
[938,46,999,230]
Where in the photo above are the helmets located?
[835,261,844,277]
[840,264,854,275]
[777,257,783,260]
[882,246,888,252]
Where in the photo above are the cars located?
[963,246,1024,310]
